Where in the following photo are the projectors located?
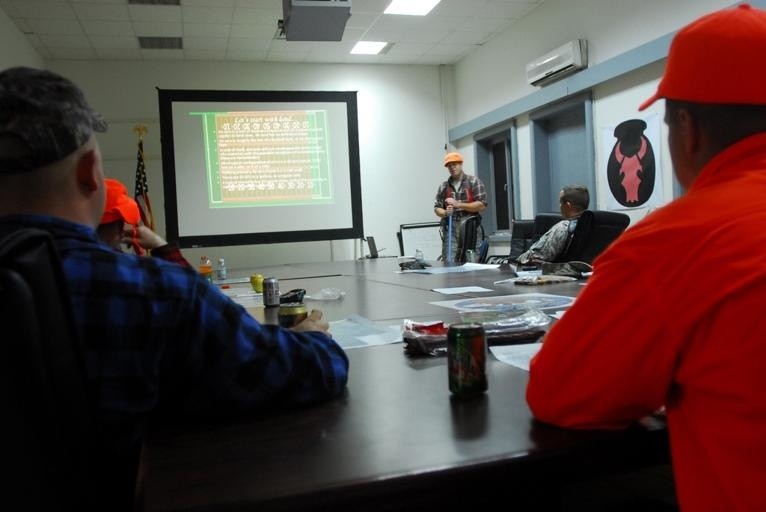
[277,0,352,41]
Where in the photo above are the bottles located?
[199,256,213,283]
[217,259,226,279]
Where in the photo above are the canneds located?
[277,302,307,329]
[466,249,475,263]
[250,274,264,293]
[263,277,280,307]
[447,321,488,396]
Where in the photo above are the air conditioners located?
[525,38,588,88]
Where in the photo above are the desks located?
[130,255,669,512]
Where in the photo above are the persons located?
[0,65,350,511]
[517,185,591,265]
[526,5,765,510]
[96,176,196,275]
[434,153,487,261]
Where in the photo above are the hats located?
[639,4,765,111]
[542,262,593,278]
[400,263,432,270]
[99,178,142,224]
[1,66,108,173]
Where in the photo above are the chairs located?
[485,211,631,267]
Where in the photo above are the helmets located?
[443,153,463,166]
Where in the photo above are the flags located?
[135,141,155,231]
